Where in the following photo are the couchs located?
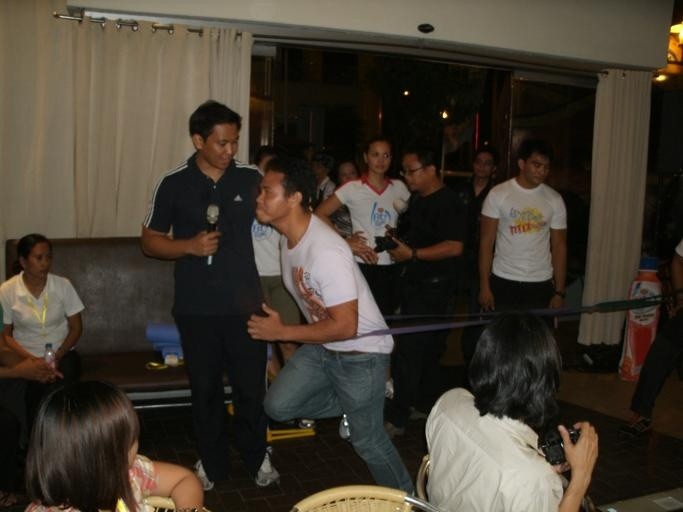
[5,237,305,356]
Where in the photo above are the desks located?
[26,354,236,449]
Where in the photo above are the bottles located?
[44,343,56,370]
[616,259,665,382]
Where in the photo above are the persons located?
[427,311,600,512]
[616,232,682,441]
[1,232,87,512]
[248,156,417,508]
[140,99,283,491]
[23,379,205,512]
[248,139,570,436]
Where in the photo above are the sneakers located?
[196,461,214,492]
[340,414,350,438]
[299,420,316,429]
[617,416,652,437]
[257,452,280,485]
[384,378,393,398]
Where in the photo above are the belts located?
[412,275,449,283]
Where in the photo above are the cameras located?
[542,425,579,468]
[374,228,406,255]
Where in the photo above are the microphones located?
[206,205,220,266]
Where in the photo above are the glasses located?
[400,166,424,176]
